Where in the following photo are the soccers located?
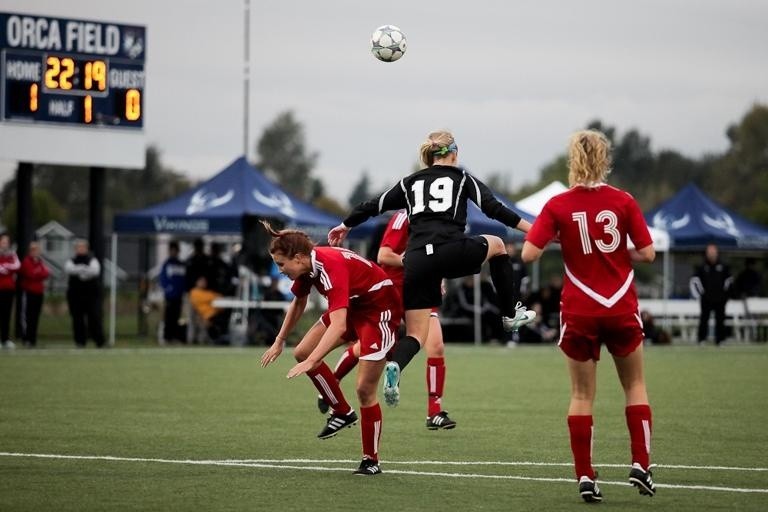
[369,25,406,62]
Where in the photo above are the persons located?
[325,126,560,410]
[156,237,295,348]
[443,257,564,347]
[515,125,659,506]
[686,241,740,351]
[640,311,671,345]
[0,226,106,350]
[314,208,459,433]
[255,217,407,478]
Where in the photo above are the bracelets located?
[275,334,288,342]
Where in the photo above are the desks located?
[209,296,315,346]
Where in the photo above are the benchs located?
[630,294,767,345]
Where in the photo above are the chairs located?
[182,292,213,345]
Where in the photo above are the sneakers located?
[425,410,456,430]
[502,301,536,333]
[383,361,400,408]
[318,392,329,413]
[318,407,359,440]
[628,462,657,496]
[352,454,382,476]
[579,471,602,503]
